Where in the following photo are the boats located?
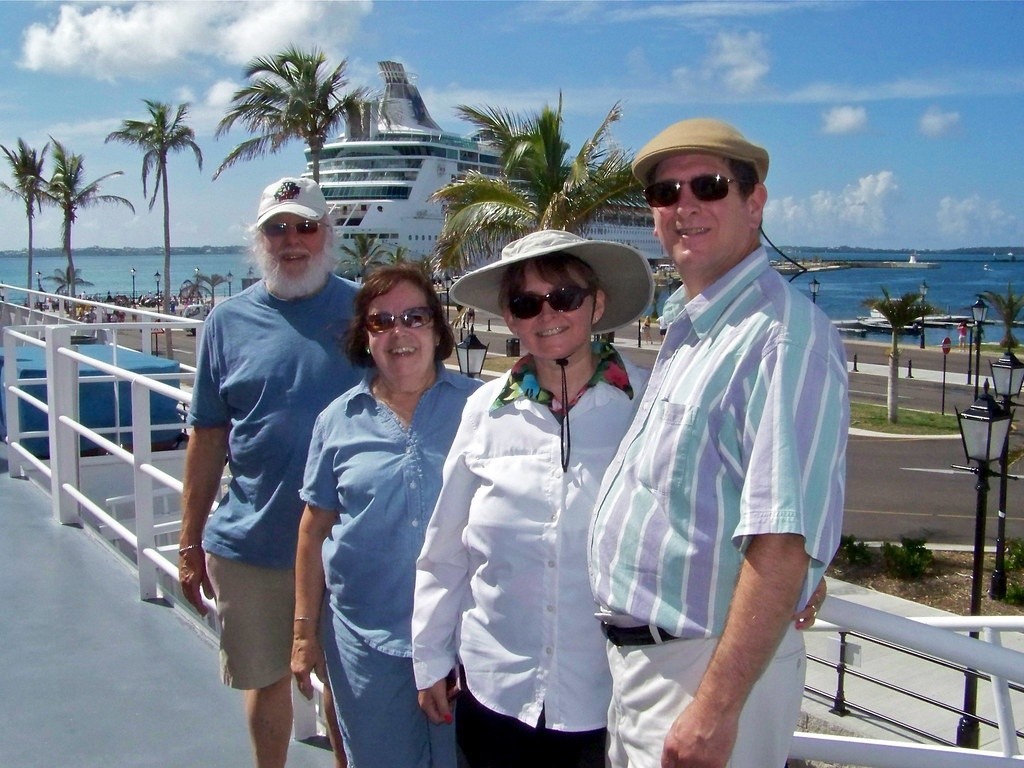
[859,318,922,335]
[870,298,972,322]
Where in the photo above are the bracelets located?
[178,545,201,554]
[294,617,310,622]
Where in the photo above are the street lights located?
[987,344,1024,600]
[154,270,161,313]
[441,273,453,323]
[226,270,233,297]
[35,270,42,290]
[104,291,115,323]
[919,280,929,348]
[667,275,674,296]
[130,268,136,302]
[808,275,821,304]
[951,378,1019,749]
[247,268,254,286]
[971,296,989,402]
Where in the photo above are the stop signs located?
[942,337,951,354]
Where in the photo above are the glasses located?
[507,285,595,319]
[363,306,434,334]
[642,174,761,208]
[261,221,332,237]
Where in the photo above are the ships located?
[301,61,673,283]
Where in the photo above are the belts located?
[599,618,675,648]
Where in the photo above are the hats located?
[632,119,769,188]
[255,176,329,228]
[448,229,656,335]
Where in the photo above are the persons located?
[179,179,366,768]
[468,308,475,324]
[412,229,828,768]
[655,313,667,345]
[958,322,967,353]
[138,290,164,308]
[47,297,59,313]
[77,291,137,323]
[170,296,178,313]
[587,118,850,768]
[290,266,486,768]
[643,316,653,345]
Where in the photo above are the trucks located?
[181,305,210,335]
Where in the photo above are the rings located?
[812,605,816,610]
[814,612,817,618]
